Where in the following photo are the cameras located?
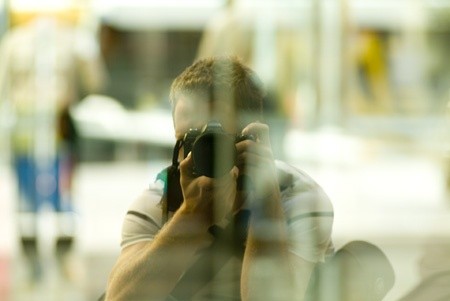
[183,123,256,182]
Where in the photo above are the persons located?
[190,0,246,72]
[0,13,99,300]
[104,58,333,301]
